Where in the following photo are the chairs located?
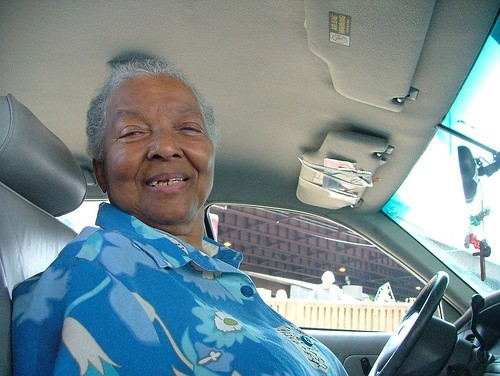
[0,93,88,376]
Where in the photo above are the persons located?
[311,271,343,303]
[13,58,347,376]
